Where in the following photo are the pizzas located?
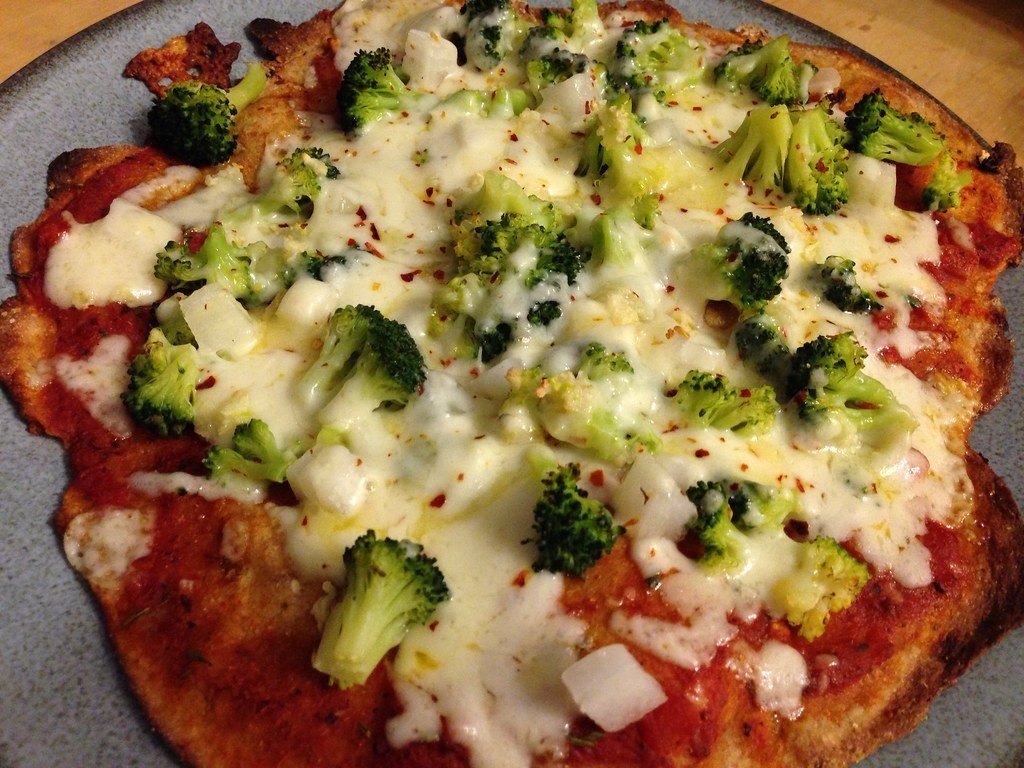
[2,2,1023,768]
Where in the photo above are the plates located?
[0,0,1024,768]
